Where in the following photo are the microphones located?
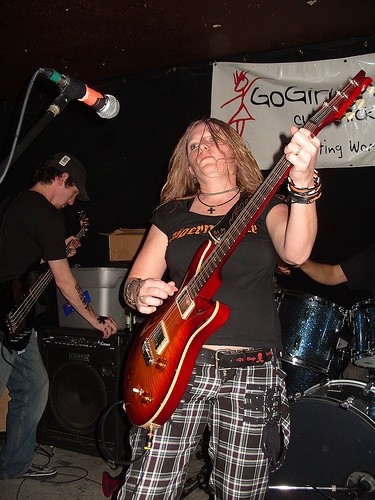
[45,68,120,119]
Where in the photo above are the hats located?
[44,152,88,196]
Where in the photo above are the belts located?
[196,347,272,370]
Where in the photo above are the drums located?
[263,376,374,499]
[344,296,373,368]
[272,287,350,376]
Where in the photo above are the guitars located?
[120,69,373,431]
[0,210,90,350]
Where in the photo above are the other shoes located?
[19,463,57,477]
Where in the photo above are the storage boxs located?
[57,267,127,330]
[108,228,146,261]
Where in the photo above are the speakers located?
[36,323,141,462]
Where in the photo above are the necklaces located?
[199,186,239,197]
[196,190,242,215]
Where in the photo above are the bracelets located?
[285,170,322,205]
[125,278,141,306]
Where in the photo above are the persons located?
[115,118,321,500]
[277,248,375,291]
[0,154,118,480]
